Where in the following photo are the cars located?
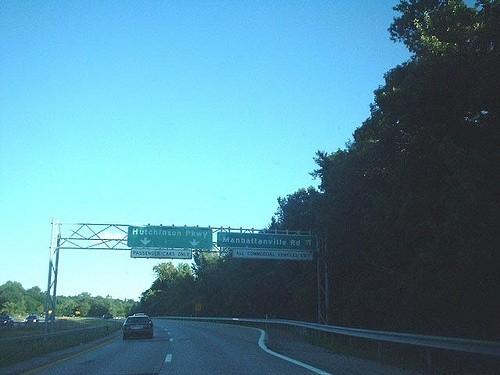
[0,314,14,330]
[102,313,114,319]
[26,316,40,323]
[122,313,153,339]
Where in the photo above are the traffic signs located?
[128,226,212,251]
